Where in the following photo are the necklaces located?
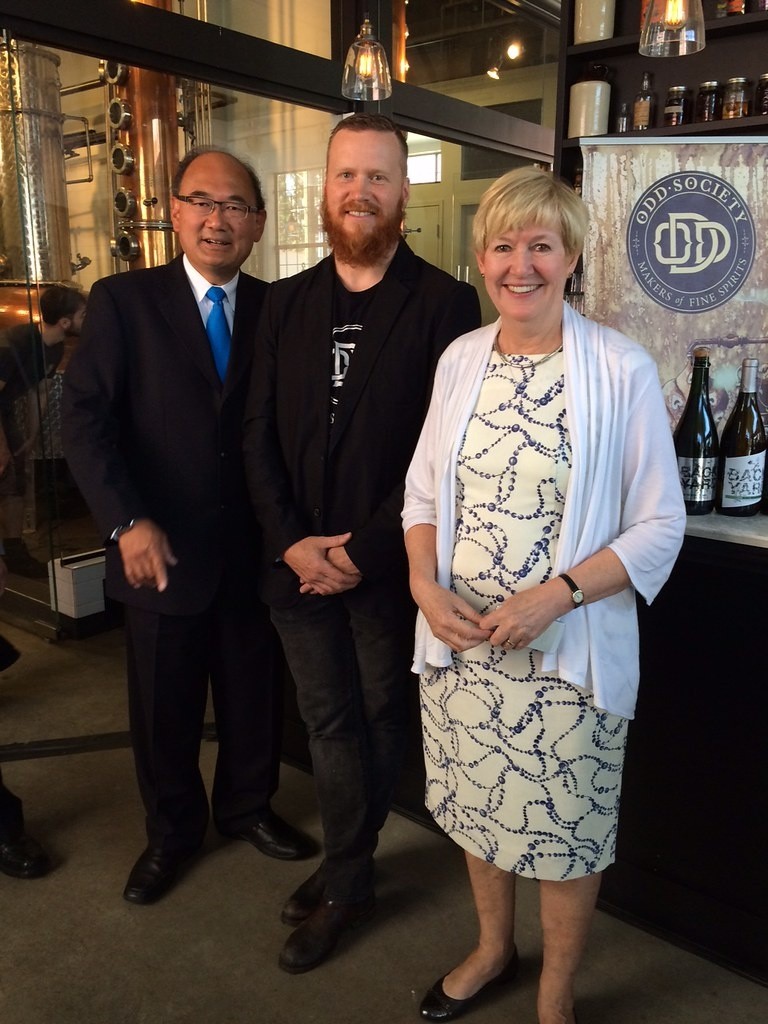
[494,336,563,368]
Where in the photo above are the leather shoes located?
[233,810,304,859]
[0,835,48,877]
[278,893,378,975]
[281,859,376,926]
[419,942,518,1022]
[124,837,202,904]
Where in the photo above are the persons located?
[0,767,50,879]
[62,152,314,905]
[0,287,86,575]
[239,113,482,975]
[400,166,686,1024]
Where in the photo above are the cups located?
[588,65,608,80]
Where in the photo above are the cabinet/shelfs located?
[553,0,768,315]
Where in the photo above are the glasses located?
[175,194,258,220]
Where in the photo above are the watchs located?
[559,574,584,609]
[120,519,134,533]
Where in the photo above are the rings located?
[507,640,515,646]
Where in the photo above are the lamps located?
[339,17,392,102]
[638,0,706,58]
[486,34,523,79]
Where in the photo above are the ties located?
[206,286,231,386]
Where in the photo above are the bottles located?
[697,81,722,122]
[714,358,768,517]
[616,102,631,133]
[716,0,745,18]
[722,77,752,119]
[673,348,719,513]
[632,72,653,130]
[664,86,687,126]
[756,73,768,116]
